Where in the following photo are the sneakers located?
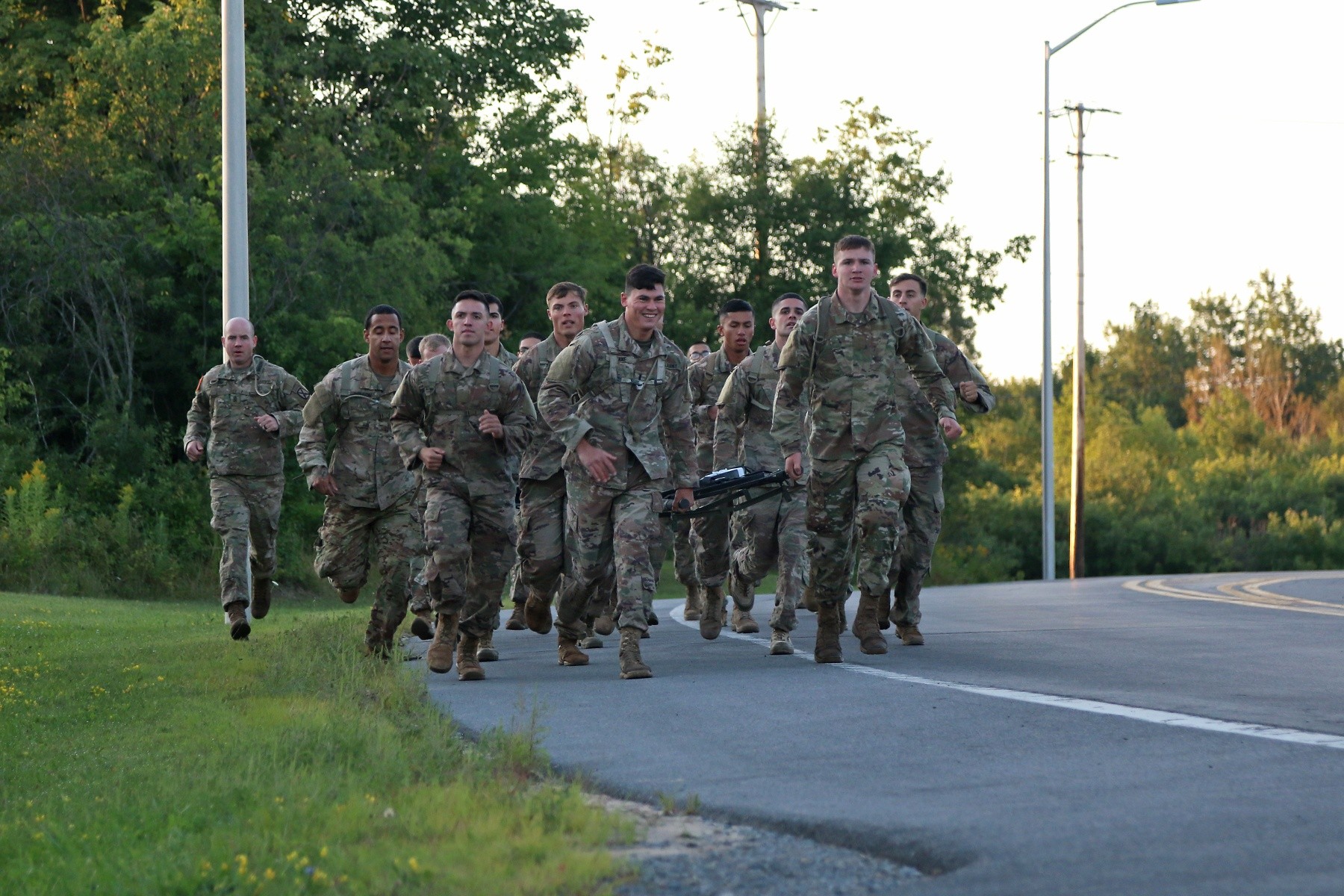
[336,586,359,603]
[771,628,794,654]
[804,585,819,613]
[577,628,603,649]
[837,600,847,631]
[524,590,552,634]
[730,567,756,611]
[593,591,617,635]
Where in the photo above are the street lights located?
[1041,1,1196,583]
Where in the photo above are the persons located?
[389,290,538,680]
[183,317,311,640]
[769,235,963,663]
[294,273,996,665]
[537,263,701,678]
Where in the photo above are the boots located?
[877,587,891,630]
[556,635,589,665]
[699,585,723,639]
[226,603,250,640]
[618,626,653,679]
[426,614,459,674]
[682,584,701,620]
[456,631,485,681]
[731,601,760,634]
[475,628,500,662]
[895,623,924,646]
[411,609,435,640]
[852,594,888,654]
[506,598,529,631]
[250,577,272,619]
[814,602,843,663]
[720,590,728,626]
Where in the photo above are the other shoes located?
[647,612,659,626]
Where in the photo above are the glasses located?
[688,352,711,360]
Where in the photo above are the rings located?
[263,425,267,430]
